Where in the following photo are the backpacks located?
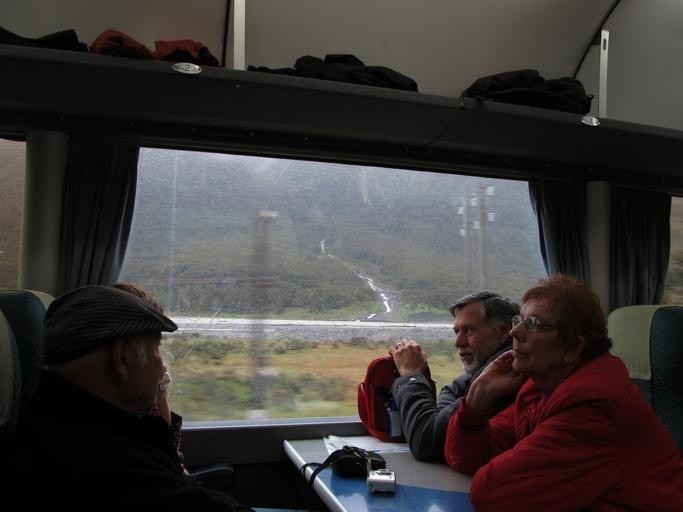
[464,70,595,115]
[247,54,419,97]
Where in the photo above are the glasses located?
[511,315,564,332]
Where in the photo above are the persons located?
[2,286,254,511]
[112,283,182,462]
[443,277,683,512]
[388,291,522,462]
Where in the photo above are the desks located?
[283,435,475,511]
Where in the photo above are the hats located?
[43,286,178,364]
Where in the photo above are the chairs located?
[0,308,23,432]
[607,304,681,453]
[0,289,57,375]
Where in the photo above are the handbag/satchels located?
[301,445,386,487]
[358,357,436,442]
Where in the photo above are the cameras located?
[367,469,395,495]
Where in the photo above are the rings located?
[396,341,403,348]
